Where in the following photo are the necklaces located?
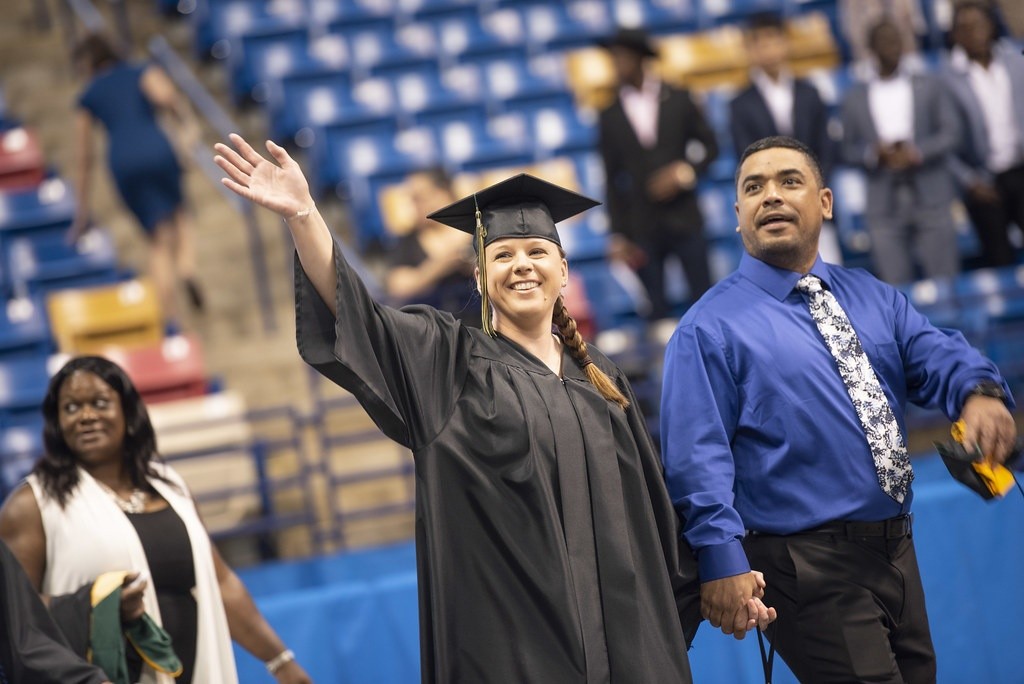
[100,481,145,513]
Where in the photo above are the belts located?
[742,511,913,540]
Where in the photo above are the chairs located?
[0,0,1024,567]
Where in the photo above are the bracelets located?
[266,649,295,672]
[283,200,315,223]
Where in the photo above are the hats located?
[592,27,659,59]
[425,172,603,339]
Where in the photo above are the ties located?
[795,273,915,504]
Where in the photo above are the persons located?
[659,136,1017,684]
[0,355,312,684]
[732,14,845,276]
[590,28,718,317]
[68,30,204,331]
[837,0,1023,283]
[213,130,776,684]
